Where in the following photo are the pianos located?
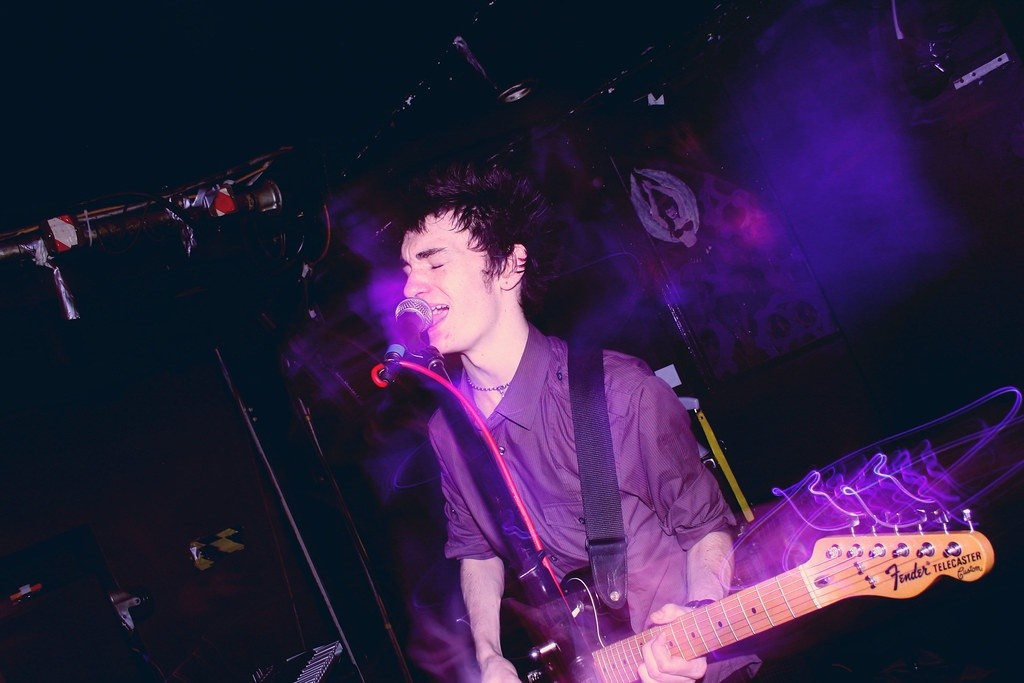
[223,637,343,683]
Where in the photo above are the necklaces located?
[466,377,509,396]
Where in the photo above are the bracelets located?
[685,599,716,609]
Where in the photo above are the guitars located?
[502,507,995,683]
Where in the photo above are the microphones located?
[381,297,432,383]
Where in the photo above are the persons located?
[399,161,763,683]
